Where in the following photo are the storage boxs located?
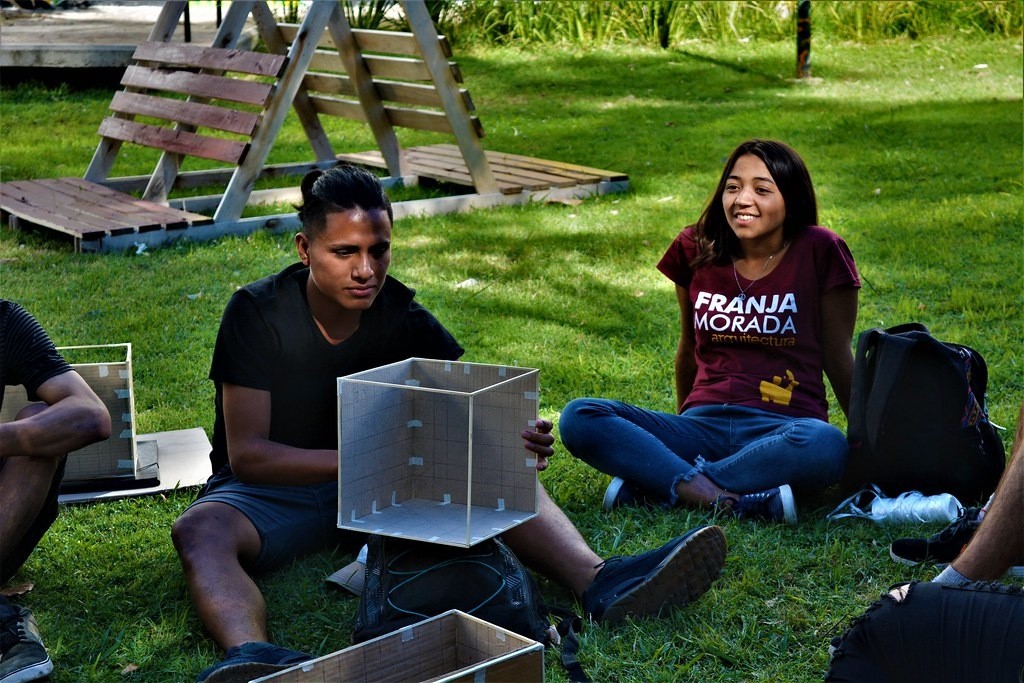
[247,607,546,683]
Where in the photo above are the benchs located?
[0,0,630,254]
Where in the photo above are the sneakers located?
[0,582,54,683]
[581,524,728,631]
[196,641,319,683]
[602,477,650,513]
[711,484,798,530]
[890,507,982,569]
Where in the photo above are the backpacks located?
[350,533,589,683]
[849,323,1007,506]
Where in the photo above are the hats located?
[325,543,379,596]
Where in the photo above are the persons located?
[0,301,111,683]
[171,161,727,683]
[824,404,1024,683]
[558,138,862,526]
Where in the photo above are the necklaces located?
[734,256,772,301]
